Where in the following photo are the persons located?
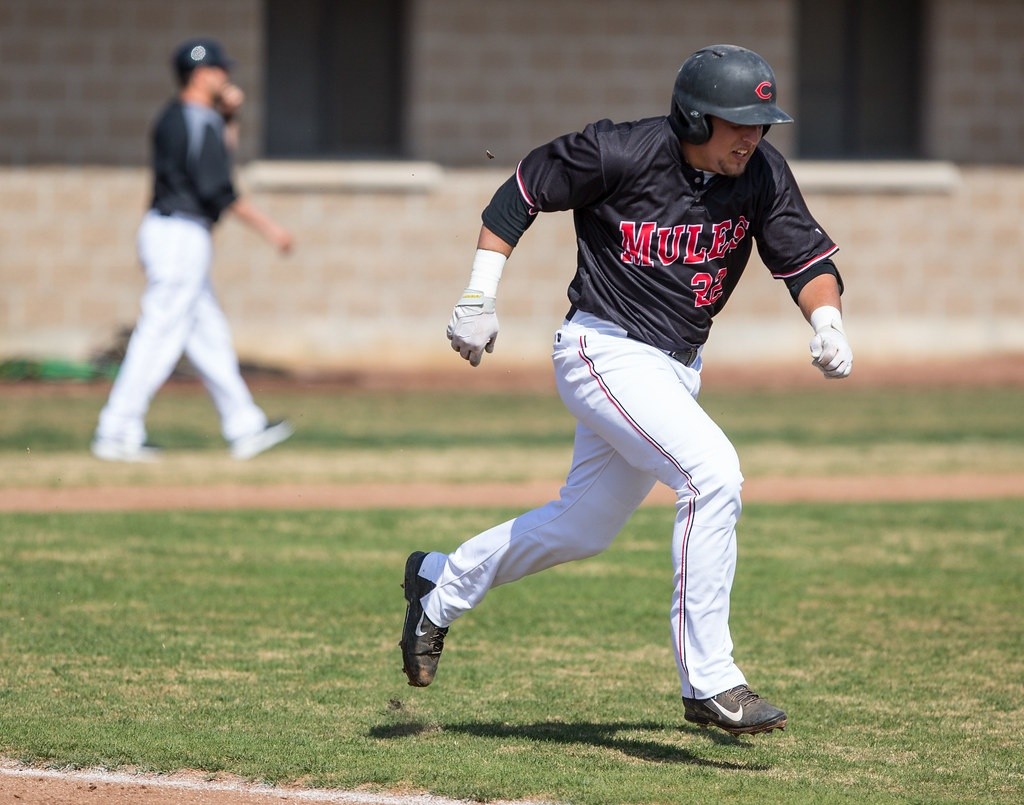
[87,36,297,462]
[398,46,854,735]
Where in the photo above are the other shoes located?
[231,420,291,460]
[91,437,162,461]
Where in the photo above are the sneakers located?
[681,684,788,738]
[399,551,450,688]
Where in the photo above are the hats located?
[171,38,232,71]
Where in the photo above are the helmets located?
[669,46,794,145]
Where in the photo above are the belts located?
[565,304,697,367]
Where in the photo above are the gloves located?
[446,288,499,367]
[810,323,853,379]
